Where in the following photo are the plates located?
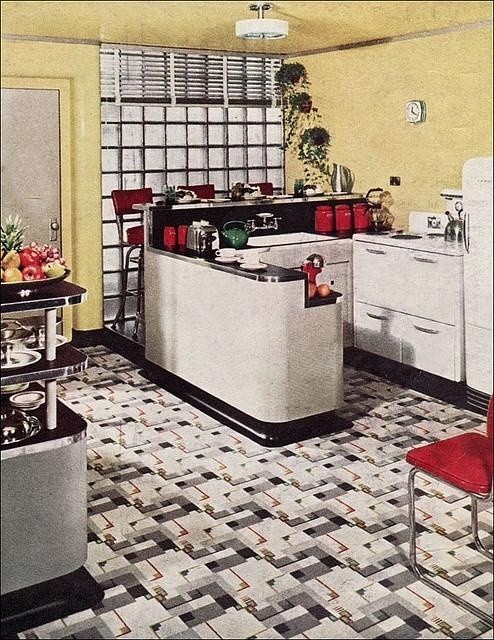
[243,194,266,199]
[1,327,68,373]
[177,197,201,203]
[215,257,238,263]
[214,248,236,259]
[0,382,45,411]
[208,198,231,202]
[240,263,268,269]
[1,269,71,294]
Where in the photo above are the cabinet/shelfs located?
[352,241,464,406]
[265,240,353,365]
[0,280,105,634]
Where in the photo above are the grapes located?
[30,242,66,265]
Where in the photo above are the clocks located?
[406,99,425,122]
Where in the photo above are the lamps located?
[235,4,287,40]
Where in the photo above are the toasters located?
[187,224,220,252]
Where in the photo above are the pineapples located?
[1,213,30,261]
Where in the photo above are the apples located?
[23,265,46,281]
[19,246,42,269]
[309,283,316,299]
[317,283,330,298]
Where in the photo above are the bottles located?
[294,178,305,198]
[314,203,369,234]
[163,225,189,247]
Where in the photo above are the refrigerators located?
[462,157,493,417]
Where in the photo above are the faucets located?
[247,212,281,232]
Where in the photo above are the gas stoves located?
[352,229,462,256]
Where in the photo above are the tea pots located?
[222,219,253,247]
[444,211,464,244]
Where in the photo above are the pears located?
[43,262,66,279]
[1,267,23,283]
[2,250,21,270]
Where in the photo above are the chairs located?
[407,395,494,624]
[111,188,153,337]
[236,182,274,196]
[177,185,215,198]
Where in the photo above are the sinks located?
[247,232,338,247]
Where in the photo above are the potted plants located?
[297,126,331,186]
[273,61,309,102]
[279,90,312,152]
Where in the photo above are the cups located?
[237,253,260,265]
